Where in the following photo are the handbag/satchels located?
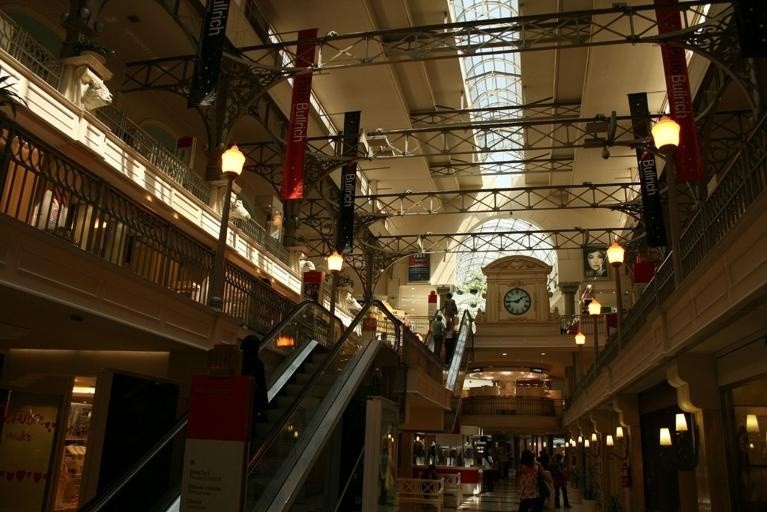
[536,472,551,500]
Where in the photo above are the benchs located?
[420,472,463,507]
[396,478,445,511]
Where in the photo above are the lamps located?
[564,413,698,471]
[745,414,761,437]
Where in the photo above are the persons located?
[436,293,456,329]
[441,321,455,365]
[412,440,572,511]
[429,315,445,357]
[584,247,608,280]
[238,333,269,454]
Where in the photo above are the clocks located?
[504,288,531,315]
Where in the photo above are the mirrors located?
[734,404,766,468]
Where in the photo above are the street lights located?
[210,143,246,311]
[574,333,585,378]
[324,251,343,347]
[587,299,602,360]
[606,241,625,335]
